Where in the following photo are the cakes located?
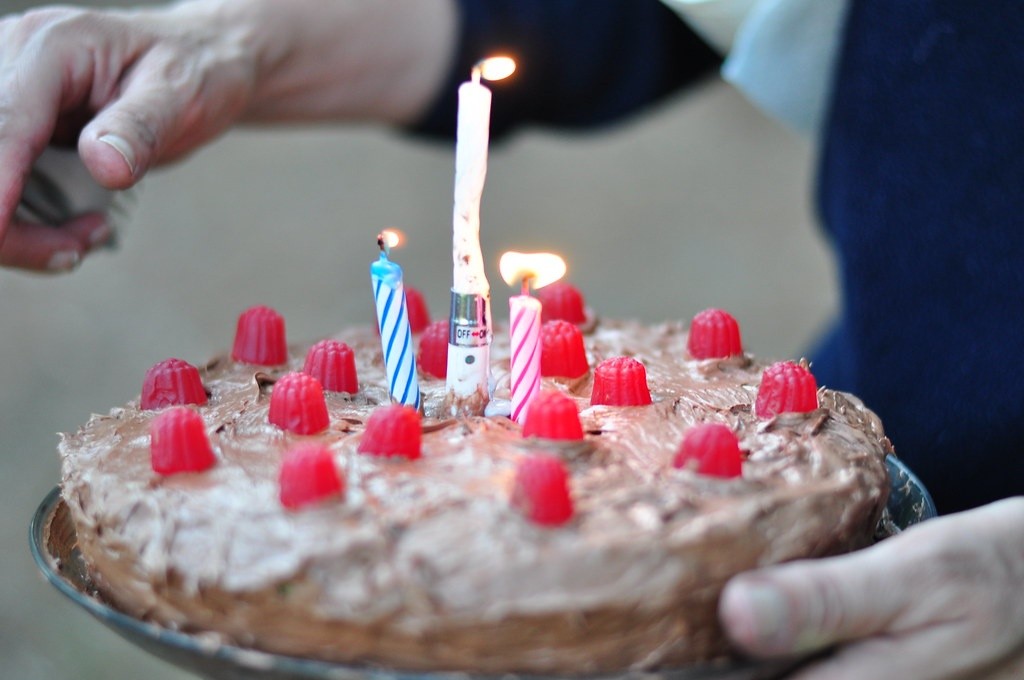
[57,279,890,674]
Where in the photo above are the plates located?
[28,456,936,680]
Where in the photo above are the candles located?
[453,56,517,295]
[370,229,425,416]
[498,250,567,423]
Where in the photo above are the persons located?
[0,1,1023,679]
